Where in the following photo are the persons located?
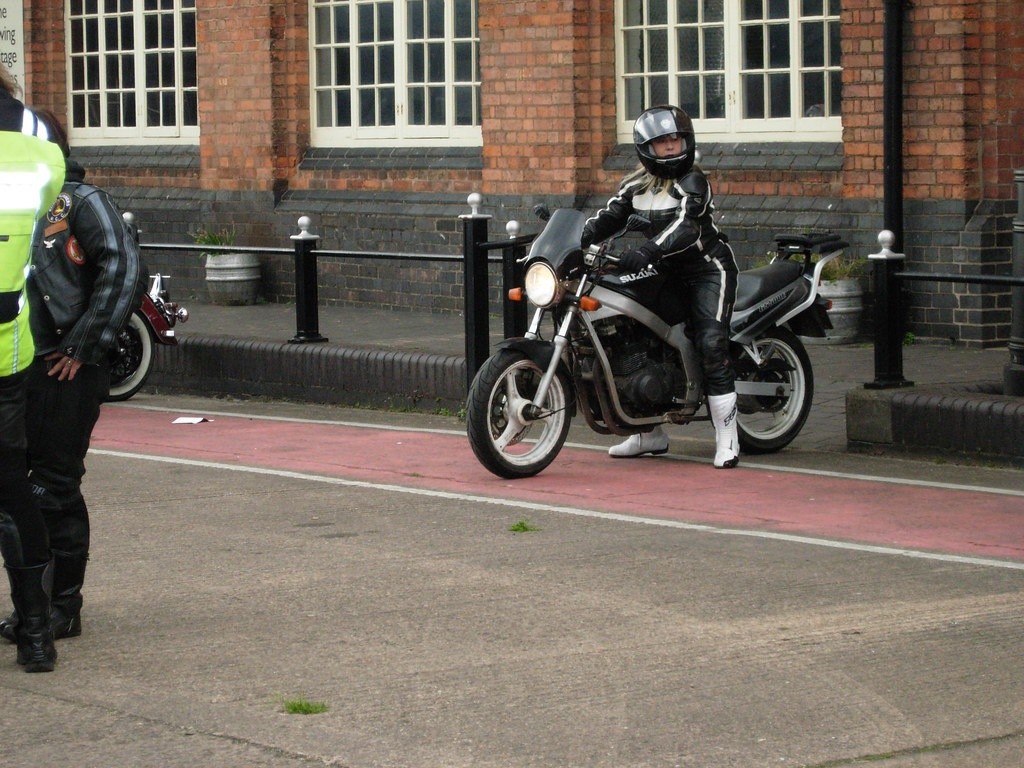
[1,63,68,673]
[0,103,141,643]
[531,102,743,468]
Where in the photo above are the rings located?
[66,361,74,367]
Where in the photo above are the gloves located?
[619,241,667,280]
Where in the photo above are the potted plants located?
[186,224,262,305]
[754,227,871,344]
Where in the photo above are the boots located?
[3,551,58,673]
[608,426,670,458]
[708,392,740,468]
[0,549,89,641]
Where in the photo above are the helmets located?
[633,105,695,179]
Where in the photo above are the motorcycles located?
[464,205,849,479]
[106,210,189,411]
